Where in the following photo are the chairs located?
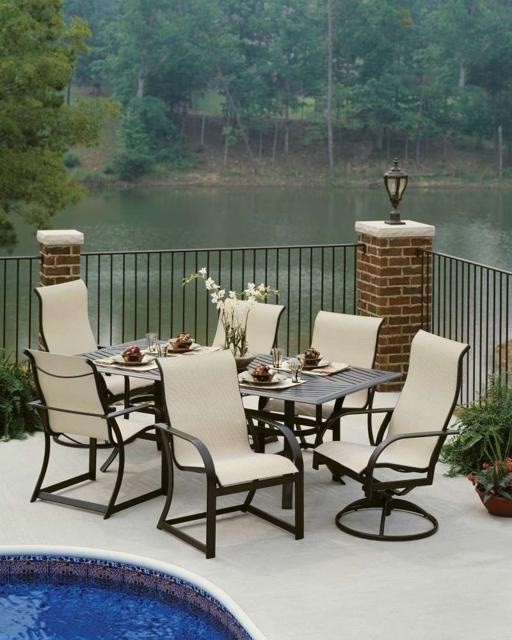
[153,349,304,559]
[313,329,471,542]
[210,298,289,448]
[258,310,386,450]
[34,275,162,453]
[21,347,172,520]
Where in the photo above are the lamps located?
[383,157,409,225]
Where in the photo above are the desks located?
[71,333,403,509]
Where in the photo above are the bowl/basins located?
[120,352,146,362]
[297,356,322,365]
[250,370,276,381]
[167,338,196,349]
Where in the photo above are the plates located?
[163,343,200,353]
[289,357,330,369]
[112,354,156,366]
[241,372,283,384]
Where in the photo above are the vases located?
[234,353,257,369]
[475,486,511,517]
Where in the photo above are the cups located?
[146,333,168,357]
[290,361,303,383]
[271,348,284,369]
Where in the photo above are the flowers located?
[179,264,280,357]
[466,456,512,503]
